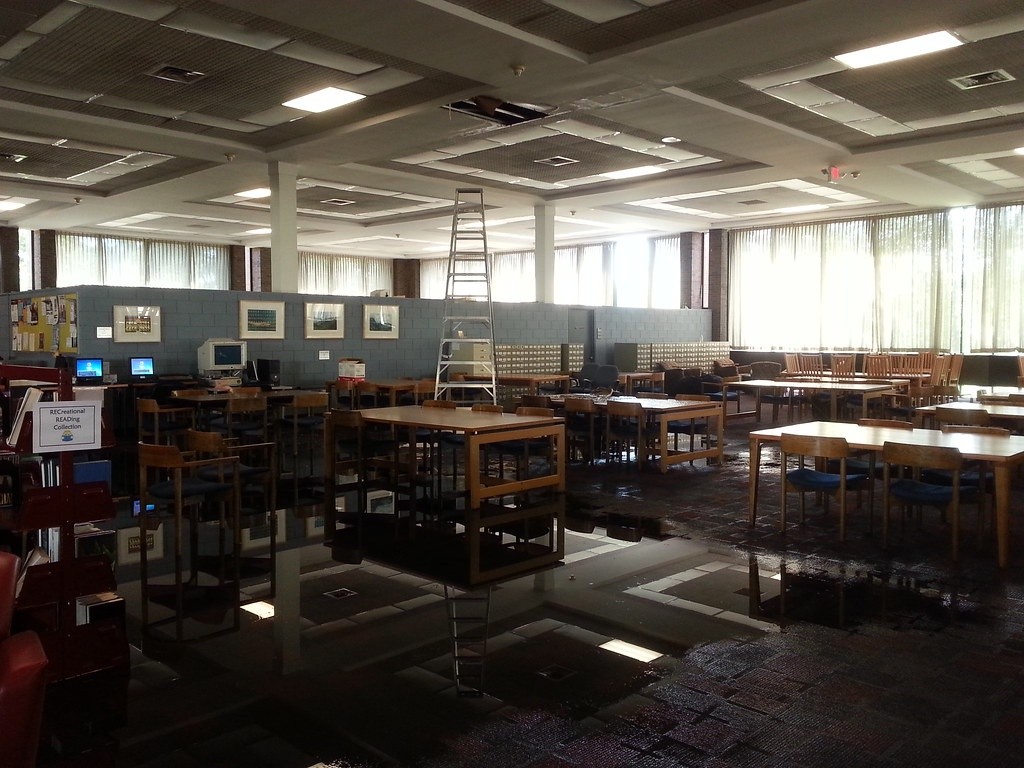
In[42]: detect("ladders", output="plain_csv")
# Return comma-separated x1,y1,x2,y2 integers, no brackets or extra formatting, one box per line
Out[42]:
433,187,505,406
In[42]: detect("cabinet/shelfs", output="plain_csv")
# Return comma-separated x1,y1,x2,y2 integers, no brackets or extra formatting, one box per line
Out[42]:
35,668,132,768
0,362,130,696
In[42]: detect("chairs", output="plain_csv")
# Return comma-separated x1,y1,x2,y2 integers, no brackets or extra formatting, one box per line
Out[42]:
182,516,276,625
139,349,1024,555
141,539,240,647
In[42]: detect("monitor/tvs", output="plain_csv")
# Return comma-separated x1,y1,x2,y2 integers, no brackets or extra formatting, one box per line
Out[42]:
75,359,103,381
129,358,154,379
197,341,247,372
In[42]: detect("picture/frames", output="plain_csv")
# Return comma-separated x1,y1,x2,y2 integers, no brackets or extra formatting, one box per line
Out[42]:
304,496,346,539
367,489,401,518
362,304,401,340
303,301,345,340
239,509,287,553
113,304,161,342
116,522,164,568
239,301,285,340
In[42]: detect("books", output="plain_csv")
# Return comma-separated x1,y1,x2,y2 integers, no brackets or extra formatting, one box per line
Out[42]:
1,450,125,636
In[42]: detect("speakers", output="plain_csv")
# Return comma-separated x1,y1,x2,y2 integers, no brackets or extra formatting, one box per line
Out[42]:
257,359,280,387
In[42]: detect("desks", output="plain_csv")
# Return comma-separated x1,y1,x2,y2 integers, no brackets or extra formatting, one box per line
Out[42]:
323,405,566,510
325,378,430,432
723,379,895,431
748,422,1024,569
915,401,1024,490
512,393,724,475
556,371,652,392
463,373,570,395
783,371,946,408
170,390,329,470
748,554,943,633
9,377,76,429
323,492,566,593
981,393,1024,405
40,383,128,402
